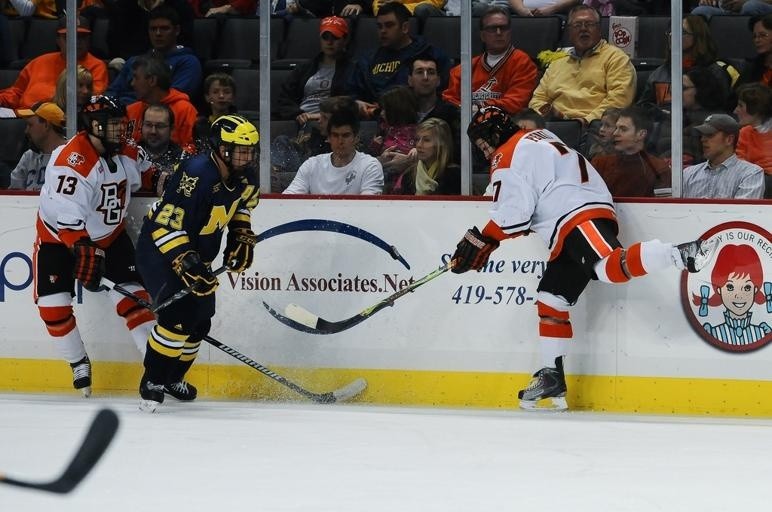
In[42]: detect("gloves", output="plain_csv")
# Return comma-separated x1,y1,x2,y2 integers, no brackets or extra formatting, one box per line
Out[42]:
470,237,500,271
451,226,486,274
222,227,256,272
72,237,106,291
171,250,219,297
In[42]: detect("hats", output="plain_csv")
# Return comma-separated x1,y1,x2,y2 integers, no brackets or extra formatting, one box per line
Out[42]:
319,16,349,39
693,114,739,135
15,101,67,129
56,15,92,34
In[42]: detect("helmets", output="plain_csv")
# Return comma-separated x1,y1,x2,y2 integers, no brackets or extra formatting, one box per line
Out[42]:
80,94,127,149
467,106,520,162
208,116,260,182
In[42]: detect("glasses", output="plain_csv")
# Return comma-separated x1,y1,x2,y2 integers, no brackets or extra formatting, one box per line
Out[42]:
752,33,770,40
142,120,168,130
570,21,597,28
681,83,694,91
683,26,693,37
480,24,511,33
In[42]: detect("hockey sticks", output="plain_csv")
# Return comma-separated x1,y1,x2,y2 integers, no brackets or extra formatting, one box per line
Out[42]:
150,262,231,315
0,409,120,494
285,257,466,333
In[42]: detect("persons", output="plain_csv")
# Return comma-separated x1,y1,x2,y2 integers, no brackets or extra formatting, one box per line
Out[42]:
363,85,419,162
391,118,460,197
0,16,109,110
283,108,384,196
529,5,633,129
407,57,461,167
683,113,765,201
692,245,772,346
133,101,193,176
509,108,545,130
279,16,352,119
452,106,720,411
7,101,66,191
134,112,259,407
296,95,359,166
637,16,716,106
191,74,237,144
127,57,199,147
731,13,772,88
589,107,672,198
442,8,539,117
645,64,711,165
510,0,583,18
52,65,94,112
586,106,622,161
734,82,769,201
340,2,451,120
32,94,171,398
105,11,201,97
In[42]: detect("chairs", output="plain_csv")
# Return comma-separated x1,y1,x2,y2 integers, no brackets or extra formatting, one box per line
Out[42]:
251,120,299,138
628,17,671,68
0,70,21,88
350,18,421,57
0,117,28,188
509,16,562,66
234,69,295,118
192,19,218,59
587,119,657,160
29,20,110,58
710,14,761,60
543,120,581,154
209,18,285,73
560,18,609,47
422,17,483,56
304,121,377,154
286,18,351,59
0,18,29,68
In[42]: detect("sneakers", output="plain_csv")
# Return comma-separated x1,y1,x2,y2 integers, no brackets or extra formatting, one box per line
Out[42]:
517,354,568,401
672,239,703,274
163,381,198,402
139,372,165,404
69,354,92,390
